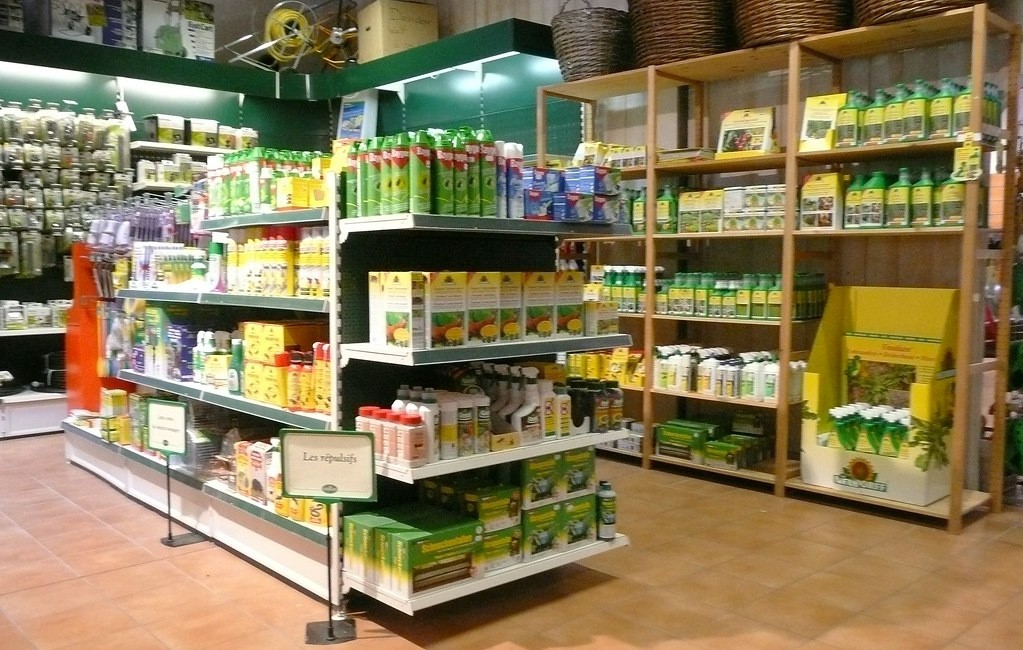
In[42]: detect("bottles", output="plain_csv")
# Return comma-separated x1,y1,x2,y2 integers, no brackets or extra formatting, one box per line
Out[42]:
355,361,623,469
601,266,825,321
623,185,677,234
285,341,330,416
208,126,524,217
844,168,985,229
192,330,245,396
655,344,807,404
834,75,1001,149
596,481,616,541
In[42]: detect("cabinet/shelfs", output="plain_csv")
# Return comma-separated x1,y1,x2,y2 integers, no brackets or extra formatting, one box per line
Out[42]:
61,208,633,639
0,140,240,438
536,5,1023,536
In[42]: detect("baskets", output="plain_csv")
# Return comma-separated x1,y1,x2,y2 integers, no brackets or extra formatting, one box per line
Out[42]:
550,0,632,83
627,0,732,70
855,0,1008,26
732,0,851,50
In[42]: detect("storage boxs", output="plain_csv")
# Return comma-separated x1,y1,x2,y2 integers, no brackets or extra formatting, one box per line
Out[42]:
653,412,771,470
365,270,619,350
356,0,439,65
341,441,600,599
240,319,329,409
